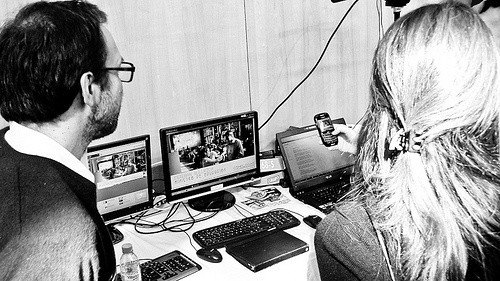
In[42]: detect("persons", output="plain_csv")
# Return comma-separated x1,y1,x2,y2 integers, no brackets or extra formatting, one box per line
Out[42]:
180,123,254,168
315,0,500,281
0,0,135,281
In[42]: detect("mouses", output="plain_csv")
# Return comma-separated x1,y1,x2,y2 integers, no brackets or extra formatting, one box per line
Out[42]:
303,215,323,229
196,248,222,263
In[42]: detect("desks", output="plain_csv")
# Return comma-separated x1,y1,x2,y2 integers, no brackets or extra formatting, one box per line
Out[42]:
112,184,328,281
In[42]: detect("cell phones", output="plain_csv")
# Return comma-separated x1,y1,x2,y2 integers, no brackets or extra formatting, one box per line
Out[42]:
314,113,338,146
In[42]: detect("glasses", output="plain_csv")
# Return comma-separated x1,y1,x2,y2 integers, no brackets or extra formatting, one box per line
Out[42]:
84,61,136,82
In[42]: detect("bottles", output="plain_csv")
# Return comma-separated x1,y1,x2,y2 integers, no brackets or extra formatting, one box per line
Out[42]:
120,243,142,281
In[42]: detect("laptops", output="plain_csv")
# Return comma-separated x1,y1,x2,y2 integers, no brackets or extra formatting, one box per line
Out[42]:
277,118,359,214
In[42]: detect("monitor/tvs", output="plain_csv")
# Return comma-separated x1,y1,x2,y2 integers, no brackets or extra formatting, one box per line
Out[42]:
159,109,260,212
86,136,153,245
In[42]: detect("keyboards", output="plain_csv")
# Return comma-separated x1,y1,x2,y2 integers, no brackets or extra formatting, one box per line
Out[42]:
194,211,301,249
114,251,203,281
